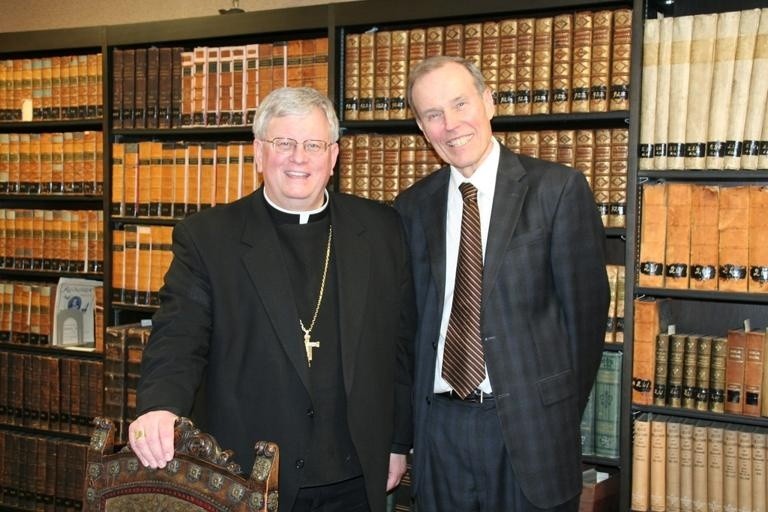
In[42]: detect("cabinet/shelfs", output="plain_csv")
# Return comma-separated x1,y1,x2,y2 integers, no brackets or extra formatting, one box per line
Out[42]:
619,0,767,512
100,1,341,418
323,1,627,509
1,23,116,511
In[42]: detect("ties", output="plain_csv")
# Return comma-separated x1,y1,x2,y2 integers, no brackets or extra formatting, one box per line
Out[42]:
441,183,486,400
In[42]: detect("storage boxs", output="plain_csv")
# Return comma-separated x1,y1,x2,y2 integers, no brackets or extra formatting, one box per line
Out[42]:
577,468,616,512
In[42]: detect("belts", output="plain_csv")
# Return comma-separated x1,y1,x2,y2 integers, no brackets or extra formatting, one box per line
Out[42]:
435,393,496,404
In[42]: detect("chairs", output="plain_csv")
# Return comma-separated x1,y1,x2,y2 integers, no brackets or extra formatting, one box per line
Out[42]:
78,411,280,512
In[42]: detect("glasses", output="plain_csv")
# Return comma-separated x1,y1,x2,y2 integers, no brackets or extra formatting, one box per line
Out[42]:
261,137,333,157
